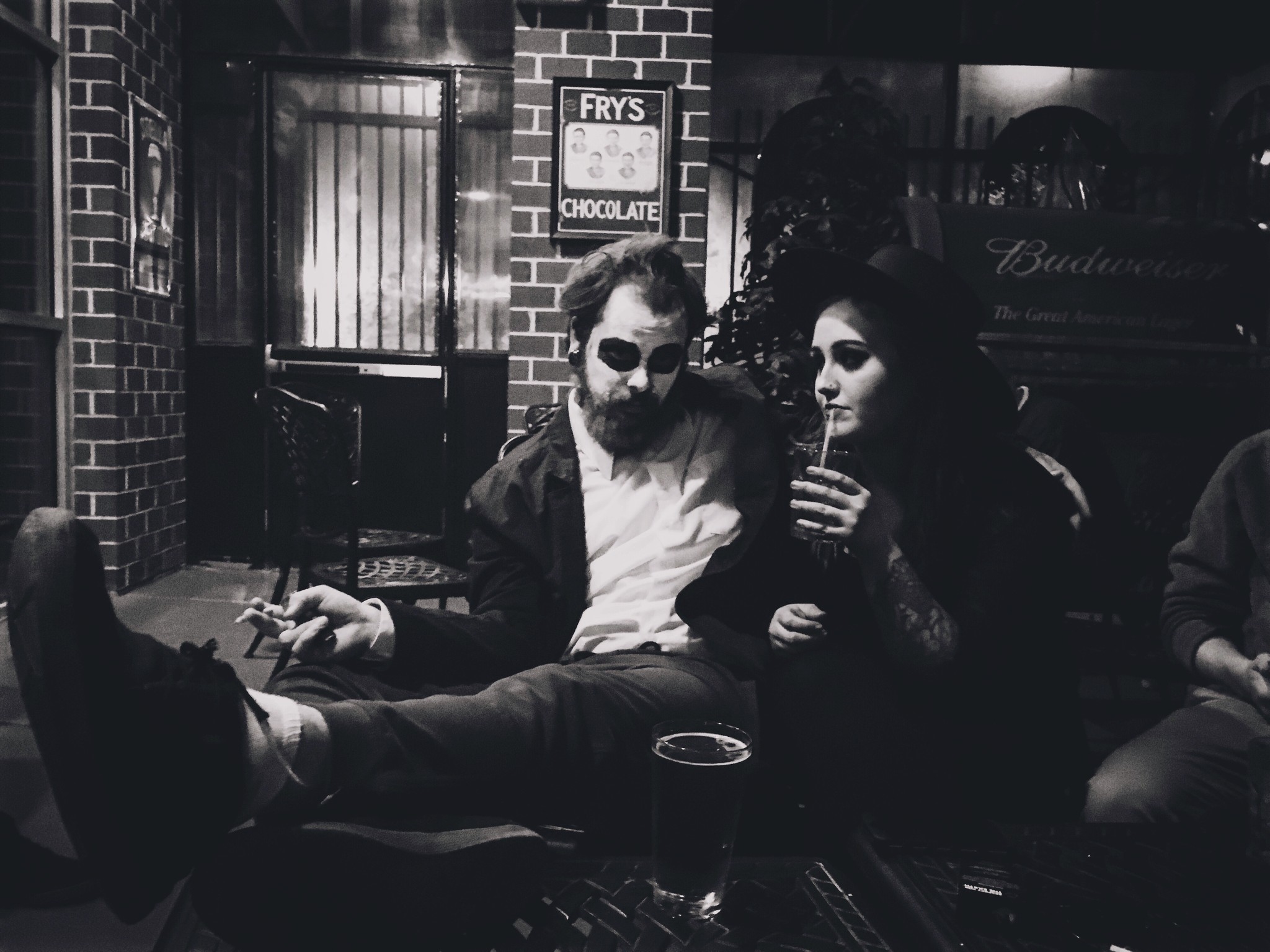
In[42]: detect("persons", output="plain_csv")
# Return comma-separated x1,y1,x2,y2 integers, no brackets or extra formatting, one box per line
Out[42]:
8,233,1090,924
571,127,655,180
673,245,1129,855
1087,414,1269,820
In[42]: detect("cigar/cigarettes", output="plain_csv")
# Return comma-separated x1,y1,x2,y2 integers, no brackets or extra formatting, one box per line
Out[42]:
232,607,273,628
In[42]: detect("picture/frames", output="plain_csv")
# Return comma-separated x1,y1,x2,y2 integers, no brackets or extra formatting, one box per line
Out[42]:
545,77,678,252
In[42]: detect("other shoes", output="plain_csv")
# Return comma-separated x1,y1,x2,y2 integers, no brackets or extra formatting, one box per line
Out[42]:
5,504,272,925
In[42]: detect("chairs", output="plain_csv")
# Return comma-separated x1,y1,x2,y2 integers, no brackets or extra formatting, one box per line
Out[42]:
263,385,473,695
236,344,450,663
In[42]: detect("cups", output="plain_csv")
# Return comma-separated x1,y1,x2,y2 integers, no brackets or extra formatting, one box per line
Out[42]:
651,719,753,920
790,447,856,564
